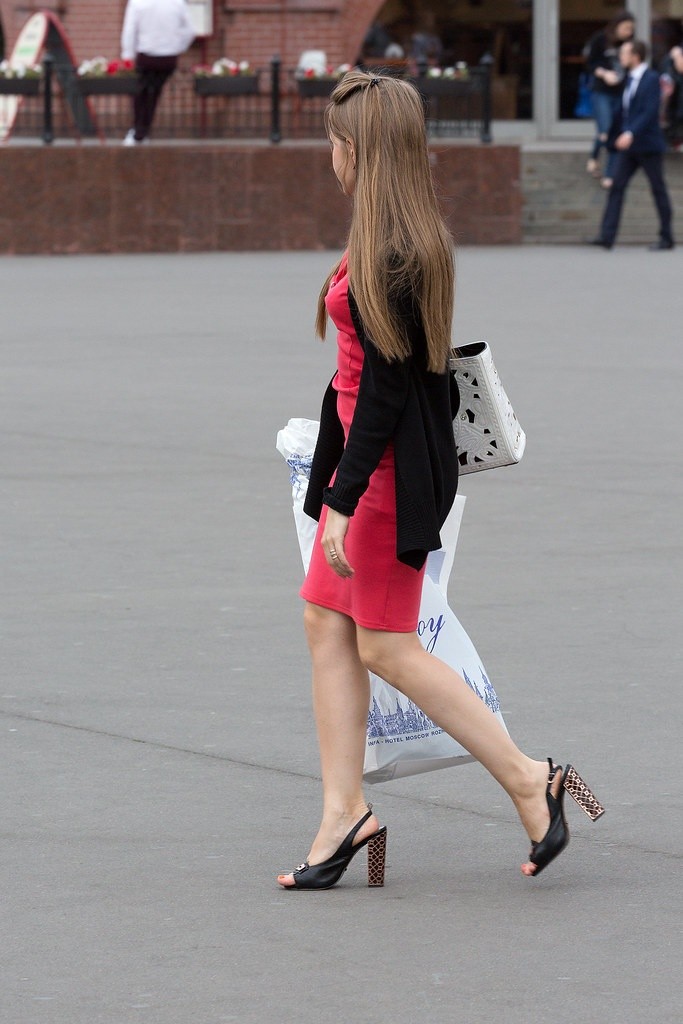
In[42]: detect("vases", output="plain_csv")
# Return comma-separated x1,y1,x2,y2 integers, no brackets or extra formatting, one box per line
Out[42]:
81,78,137,94
0,78,40,97
195,77,257,95
299,79,338,98
420,75,483,94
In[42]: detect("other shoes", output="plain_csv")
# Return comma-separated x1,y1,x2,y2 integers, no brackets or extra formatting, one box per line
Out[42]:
587,239,612,248
124,129,149,147
659,239,673,248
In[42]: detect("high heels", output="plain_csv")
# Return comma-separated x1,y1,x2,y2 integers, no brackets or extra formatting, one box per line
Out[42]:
529,758,606,876
283,802,386,890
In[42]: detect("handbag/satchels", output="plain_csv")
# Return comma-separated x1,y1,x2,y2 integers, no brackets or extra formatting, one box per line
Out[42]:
576,73,595,116
448,341,525,476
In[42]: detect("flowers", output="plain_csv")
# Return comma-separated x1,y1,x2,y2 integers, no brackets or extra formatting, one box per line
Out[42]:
185,57,255,78
73,53,138,77
0,59,45,78
425,61,471,81
291,61,351,80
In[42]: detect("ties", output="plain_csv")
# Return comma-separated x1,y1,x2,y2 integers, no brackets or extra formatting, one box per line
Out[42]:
626,77,632,90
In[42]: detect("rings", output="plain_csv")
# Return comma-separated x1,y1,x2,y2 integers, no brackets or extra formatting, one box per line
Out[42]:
330,549,338,559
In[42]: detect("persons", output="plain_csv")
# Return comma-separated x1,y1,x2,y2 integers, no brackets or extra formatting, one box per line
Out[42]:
578,13,683,188
276,69,606,889
589,40,673,250
119,0,196,146
